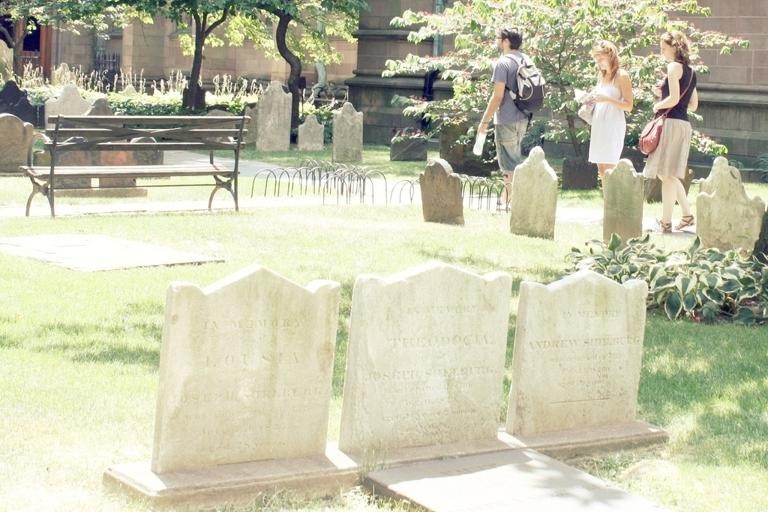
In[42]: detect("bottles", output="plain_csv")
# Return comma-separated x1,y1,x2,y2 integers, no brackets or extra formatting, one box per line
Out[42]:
472,131,488,156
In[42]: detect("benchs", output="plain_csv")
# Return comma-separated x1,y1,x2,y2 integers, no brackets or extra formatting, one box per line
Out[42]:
17,114,251,217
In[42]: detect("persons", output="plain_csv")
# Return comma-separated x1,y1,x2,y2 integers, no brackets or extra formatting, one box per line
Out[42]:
647,31,699,234
580,39,634,194
476,26,534,213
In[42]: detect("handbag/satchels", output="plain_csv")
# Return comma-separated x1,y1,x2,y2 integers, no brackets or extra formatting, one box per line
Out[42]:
637,111,667,155
577,100,597,126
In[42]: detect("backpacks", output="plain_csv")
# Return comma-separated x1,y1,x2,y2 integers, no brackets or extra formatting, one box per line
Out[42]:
499,51,546,114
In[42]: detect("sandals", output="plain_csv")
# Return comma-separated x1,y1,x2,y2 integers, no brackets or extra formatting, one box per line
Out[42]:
646,220,673,234
674,213,695,231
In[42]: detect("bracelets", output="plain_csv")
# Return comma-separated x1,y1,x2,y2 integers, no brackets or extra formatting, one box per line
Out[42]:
480,120,490,125
